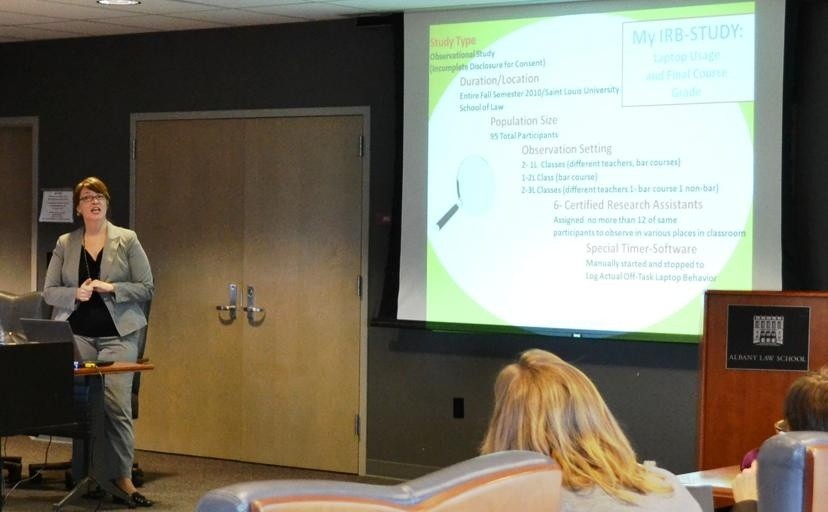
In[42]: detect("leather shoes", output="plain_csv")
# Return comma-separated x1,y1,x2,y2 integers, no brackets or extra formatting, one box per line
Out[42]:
112,483,151,506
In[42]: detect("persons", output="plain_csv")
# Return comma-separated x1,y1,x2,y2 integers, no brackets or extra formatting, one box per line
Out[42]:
44,175,156,507
479,349,705,512
730,369,828,512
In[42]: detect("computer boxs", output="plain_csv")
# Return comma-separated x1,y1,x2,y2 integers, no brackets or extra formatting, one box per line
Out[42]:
0,341,74,435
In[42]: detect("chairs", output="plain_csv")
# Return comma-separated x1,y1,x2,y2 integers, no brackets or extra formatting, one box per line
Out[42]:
1,288,152,486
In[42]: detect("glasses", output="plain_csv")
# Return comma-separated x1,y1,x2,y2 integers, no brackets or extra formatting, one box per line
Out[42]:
79,194,105,201
773,420,787,434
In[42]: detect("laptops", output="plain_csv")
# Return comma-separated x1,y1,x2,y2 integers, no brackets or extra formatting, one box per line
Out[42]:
19,318,115,368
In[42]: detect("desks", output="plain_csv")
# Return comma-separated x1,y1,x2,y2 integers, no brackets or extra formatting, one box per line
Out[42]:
48,359,154,507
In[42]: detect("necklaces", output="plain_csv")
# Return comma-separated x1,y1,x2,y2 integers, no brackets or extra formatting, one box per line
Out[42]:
82,235,90,282
